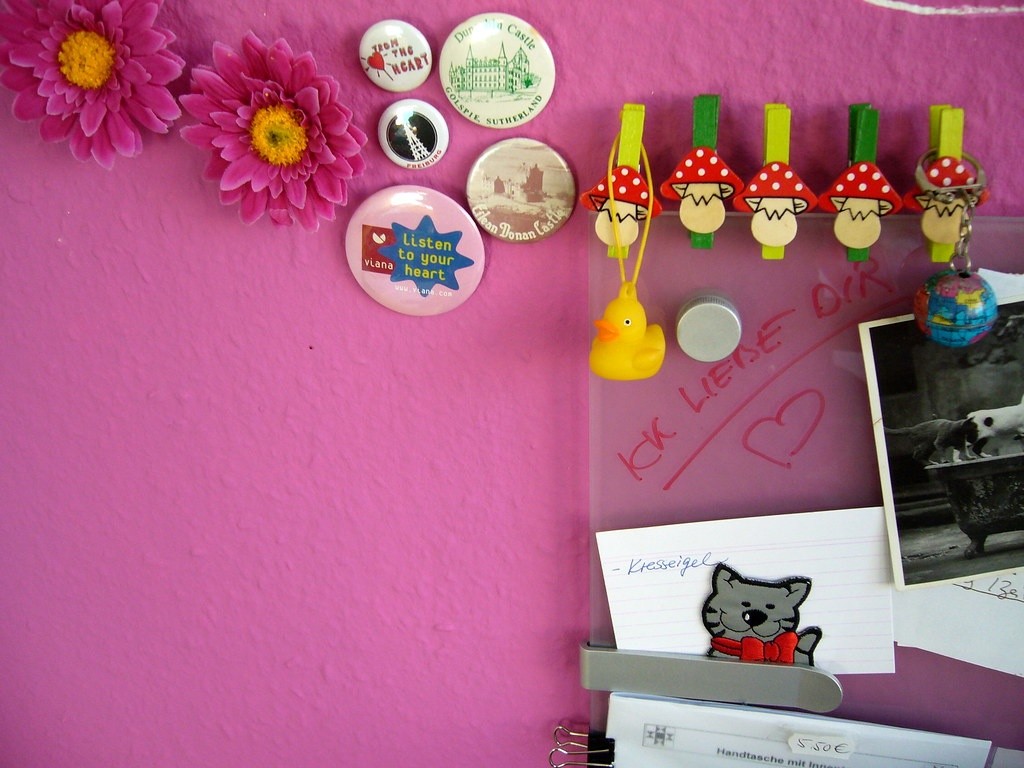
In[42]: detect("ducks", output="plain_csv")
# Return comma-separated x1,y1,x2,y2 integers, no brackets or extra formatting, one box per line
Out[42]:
588,298,665,382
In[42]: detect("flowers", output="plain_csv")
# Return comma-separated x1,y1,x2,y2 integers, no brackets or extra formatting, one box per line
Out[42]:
178,26,369,234
0,0,188,173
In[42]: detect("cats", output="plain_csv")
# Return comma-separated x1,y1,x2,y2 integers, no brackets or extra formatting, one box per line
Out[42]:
881,394,1024,465
